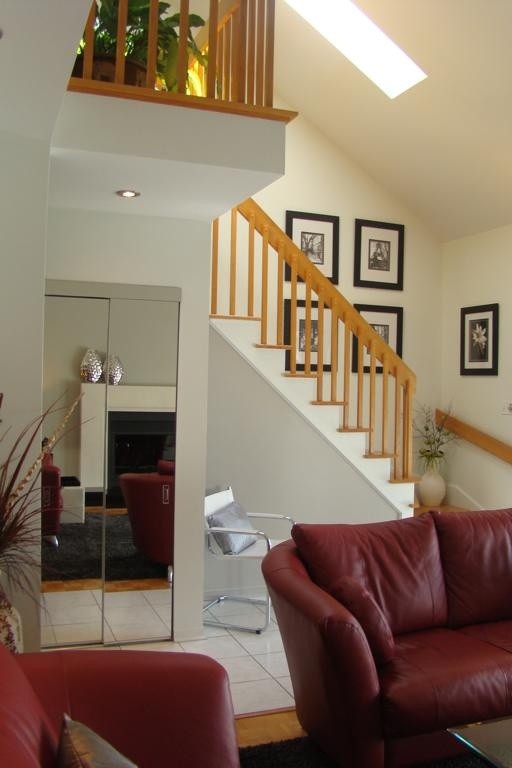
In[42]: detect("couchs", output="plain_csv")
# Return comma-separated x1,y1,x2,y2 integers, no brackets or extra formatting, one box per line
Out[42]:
250,495,511,767
0,638,238,766
111,454,174,577
39,447,65,549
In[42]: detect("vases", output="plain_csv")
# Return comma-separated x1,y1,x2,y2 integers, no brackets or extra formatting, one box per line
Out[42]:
79,347,103,383
102,354,125,386
0,595,26,653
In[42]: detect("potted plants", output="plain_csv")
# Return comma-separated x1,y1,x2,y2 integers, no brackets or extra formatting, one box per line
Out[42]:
410,403,461,507
62,1,209,101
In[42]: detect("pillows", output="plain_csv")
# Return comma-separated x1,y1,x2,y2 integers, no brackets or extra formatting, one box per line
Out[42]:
54,709,142,767
327,573,396,667
289,509,449,635
425,507,511,629
206,500,260,554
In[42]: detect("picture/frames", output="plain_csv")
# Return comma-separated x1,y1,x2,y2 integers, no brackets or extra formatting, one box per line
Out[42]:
456,298,501,376
281,295,338,374
349,300,406,374
349,215,408,293
279,207,340,287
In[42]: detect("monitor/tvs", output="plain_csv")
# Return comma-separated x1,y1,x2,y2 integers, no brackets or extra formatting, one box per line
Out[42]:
112,434,164,476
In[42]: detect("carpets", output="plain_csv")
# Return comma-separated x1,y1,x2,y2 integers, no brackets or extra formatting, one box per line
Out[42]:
43,506,163,583
238,730,501,768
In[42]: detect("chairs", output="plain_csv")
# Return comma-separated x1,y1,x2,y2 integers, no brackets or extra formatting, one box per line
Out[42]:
203,482,297,636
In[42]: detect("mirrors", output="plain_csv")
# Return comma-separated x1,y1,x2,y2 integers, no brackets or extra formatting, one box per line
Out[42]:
43,276,182,648
